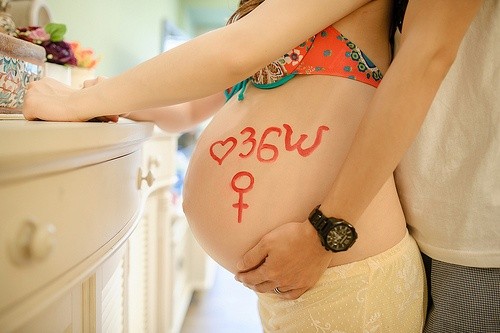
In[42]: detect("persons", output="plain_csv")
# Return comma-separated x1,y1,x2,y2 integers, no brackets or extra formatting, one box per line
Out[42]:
235,0,500,333
22,0,427,333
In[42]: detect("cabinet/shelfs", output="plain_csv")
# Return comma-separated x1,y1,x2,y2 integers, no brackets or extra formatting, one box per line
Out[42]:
0,119,213,333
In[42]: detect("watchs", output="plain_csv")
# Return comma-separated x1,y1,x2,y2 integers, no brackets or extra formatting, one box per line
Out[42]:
308,204,358,253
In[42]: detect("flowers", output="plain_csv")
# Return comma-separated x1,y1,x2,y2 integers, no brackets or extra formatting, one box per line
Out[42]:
68,40,102,68
17,22,77,65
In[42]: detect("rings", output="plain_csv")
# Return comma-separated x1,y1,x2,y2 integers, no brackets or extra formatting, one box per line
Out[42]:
273,288,281,295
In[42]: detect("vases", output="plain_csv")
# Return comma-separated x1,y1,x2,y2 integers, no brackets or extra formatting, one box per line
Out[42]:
70,67,93,89
47,64,72,88
